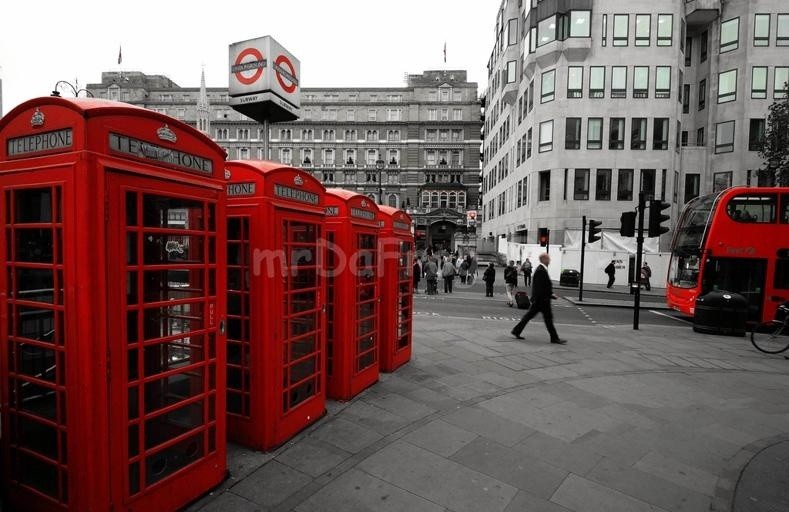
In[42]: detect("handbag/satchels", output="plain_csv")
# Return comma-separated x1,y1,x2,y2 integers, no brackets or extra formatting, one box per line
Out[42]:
482,275,487,282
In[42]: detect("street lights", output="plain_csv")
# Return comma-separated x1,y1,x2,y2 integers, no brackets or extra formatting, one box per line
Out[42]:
376,159,384,205
50,76,95,98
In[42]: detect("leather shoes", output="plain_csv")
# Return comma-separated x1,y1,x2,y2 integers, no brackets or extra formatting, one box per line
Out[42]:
550,339,568,345
511,331,525,340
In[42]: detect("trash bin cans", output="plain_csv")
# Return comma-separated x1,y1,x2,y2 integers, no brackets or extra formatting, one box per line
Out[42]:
630,282,639,295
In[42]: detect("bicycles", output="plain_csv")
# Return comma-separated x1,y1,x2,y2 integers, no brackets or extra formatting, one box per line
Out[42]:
750,304,789,354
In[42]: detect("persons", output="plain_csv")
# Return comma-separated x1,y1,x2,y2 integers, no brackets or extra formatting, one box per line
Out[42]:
604,260,616,288
503,260,518,307
481,262,496,296
515,261,522,276
522,258,532,286
510,253,568,345
412,242,478,295
641,261,651,290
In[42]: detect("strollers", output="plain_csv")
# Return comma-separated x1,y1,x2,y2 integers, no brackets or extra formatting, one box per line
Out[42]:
425,278,439,294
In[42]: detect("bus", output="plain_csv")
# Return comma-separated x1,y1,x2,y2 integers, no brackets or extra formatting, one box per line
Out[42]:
666,186,789,336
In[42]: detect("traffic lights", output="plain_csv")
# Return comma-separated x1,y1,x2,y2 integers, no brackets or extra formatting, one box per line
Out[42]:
589,219,602,243
648,202,671,236
620,211,635,236
538,227,547,247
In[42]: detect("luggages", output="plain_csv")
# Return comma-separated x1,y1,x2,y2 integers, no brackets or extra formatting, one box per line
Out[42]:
515,291,531,310
